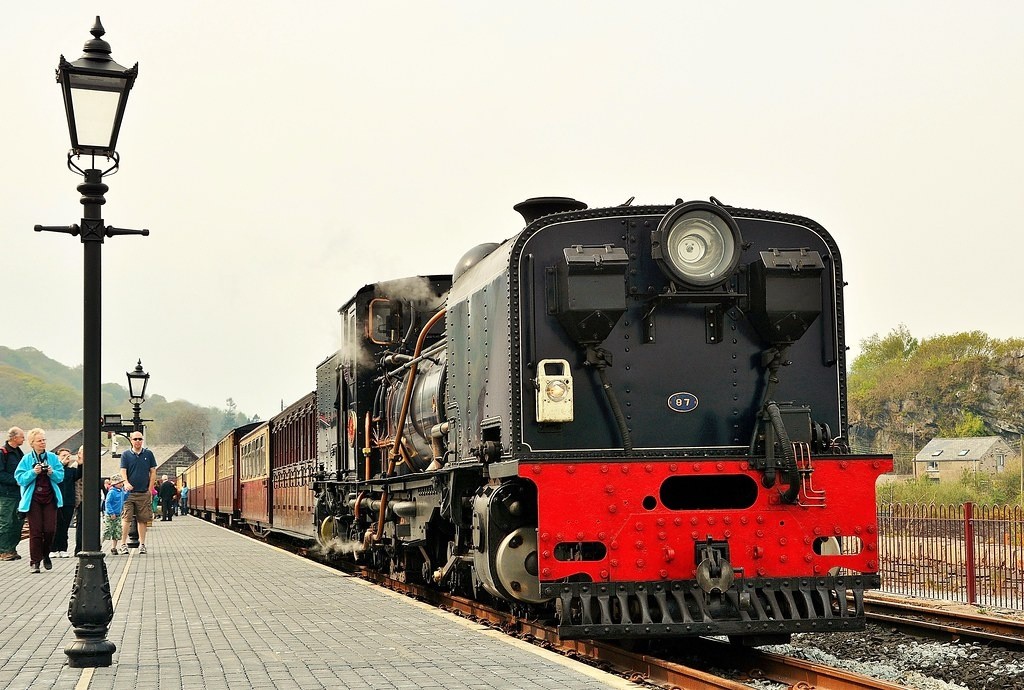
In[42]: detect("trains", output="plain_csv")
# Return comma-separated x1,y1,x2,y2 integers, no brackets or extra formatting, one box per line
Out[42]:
177,194,896,653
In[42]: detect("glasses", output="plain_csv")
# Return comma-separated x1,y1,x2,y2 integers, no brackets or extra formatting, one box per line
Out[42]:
132,438,143,441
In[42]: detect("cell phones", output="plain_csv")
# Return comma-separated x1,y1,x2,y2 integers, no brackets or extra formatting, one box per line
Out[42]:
69,455,78,460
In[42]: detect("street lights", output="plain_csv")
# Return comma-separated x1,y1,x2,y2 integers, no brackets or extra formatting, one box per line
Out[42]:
126,359,149,448
34,16,149,670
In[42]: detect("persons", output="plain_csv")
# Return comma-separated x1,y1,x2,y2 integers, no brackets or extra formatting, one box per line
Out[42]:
48,445,189,559
117,431,158,554
14,427,65,572
0,426,25,561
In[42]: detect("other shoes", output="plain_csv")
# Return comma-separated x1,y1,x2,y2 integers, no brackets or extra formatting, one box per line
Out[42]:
10,551,22,559
111,548,118,555
119,544,129,554
42,556,53,570
138,545,147,553
29,560,40,573
60,551,70,558
0,553,15,560
49,551,59,558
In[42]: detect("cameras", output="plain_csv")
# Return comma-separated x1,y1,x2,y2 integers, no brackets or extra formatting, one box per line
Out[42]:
38,452,49,472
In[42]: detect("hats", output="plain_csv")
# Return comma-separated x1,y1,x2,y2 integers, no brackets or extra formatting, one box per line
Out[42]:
110,473,126,485
161,475,168,481
